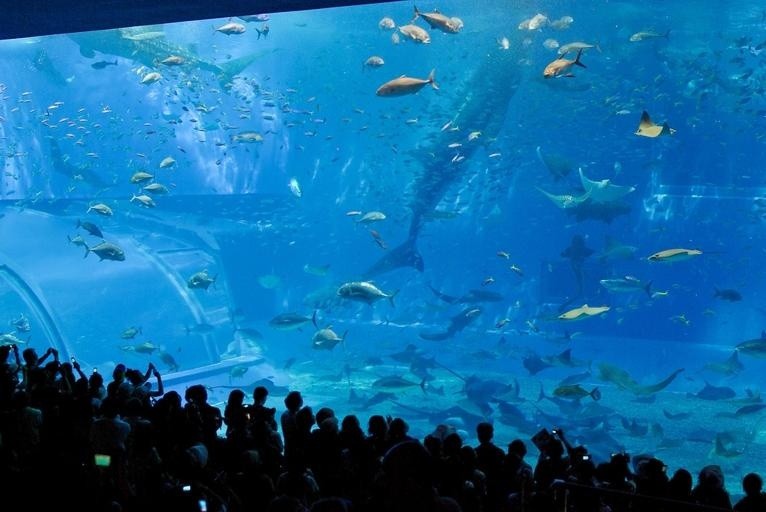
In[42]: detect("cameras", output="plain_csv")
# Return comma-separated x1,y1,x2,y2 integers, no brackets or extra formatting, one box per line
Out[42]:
552,429,558,434
94,452,112,466
182,483,192,491
582,454,592,460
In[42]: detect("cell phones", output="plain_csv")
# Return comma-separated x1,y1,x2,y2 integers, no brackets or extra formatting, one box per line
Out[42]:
198,500,207,511
242,404,249,408
71,357,76,366
93,367,98,375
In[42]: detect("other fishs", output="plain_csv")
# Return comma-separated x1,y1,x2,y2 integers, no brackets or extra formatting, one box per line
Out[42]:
0,4,766,465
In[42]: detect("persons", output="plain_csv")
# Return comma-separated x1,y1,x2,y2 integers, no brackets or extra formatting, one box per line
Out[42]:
0,344,766,512
